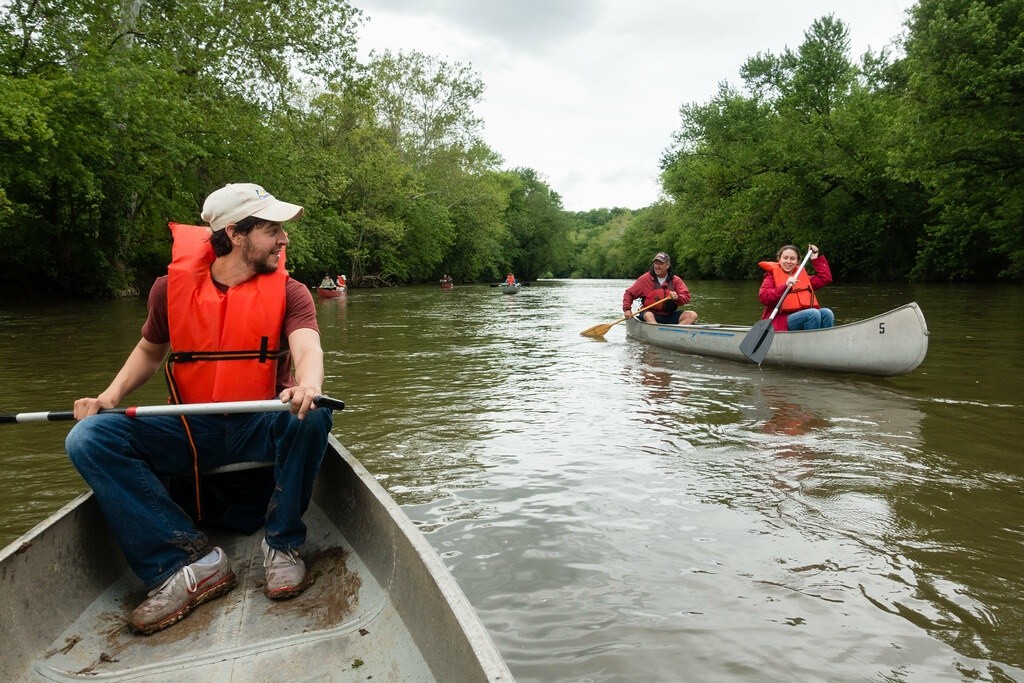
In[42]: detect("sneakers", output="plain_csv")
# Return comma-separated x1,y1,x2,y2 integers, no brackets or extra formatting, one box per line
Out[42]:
261,537,309,599
129,547,237,633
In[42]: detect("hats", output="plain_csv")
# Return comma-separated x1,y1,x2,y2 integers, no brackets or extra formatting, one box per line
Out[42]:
201,183,304,232
342,275,346,280
654,251,670,263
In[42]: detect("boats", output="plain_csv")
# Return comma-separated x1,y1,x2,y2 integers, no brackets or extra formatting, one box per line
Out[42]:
317,286,347,297
441,282,453,288
500,282,521,294
625,296,930,377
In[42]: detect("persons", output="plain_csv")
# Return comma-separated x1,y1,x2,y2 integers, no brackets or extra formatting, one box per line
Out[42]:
758,243,834,332
319,273,336,288
335,275,347,291
504,271,518,286
623,252,698,324
66,182,334,637
440,274,452,285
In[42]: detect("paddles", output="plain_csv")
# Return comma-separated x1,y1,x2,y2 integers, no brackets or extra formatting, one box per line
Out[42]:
490,282,530,287
0,395,345,424
739,247,819,364
309,286,319,288
580,294,672,337
